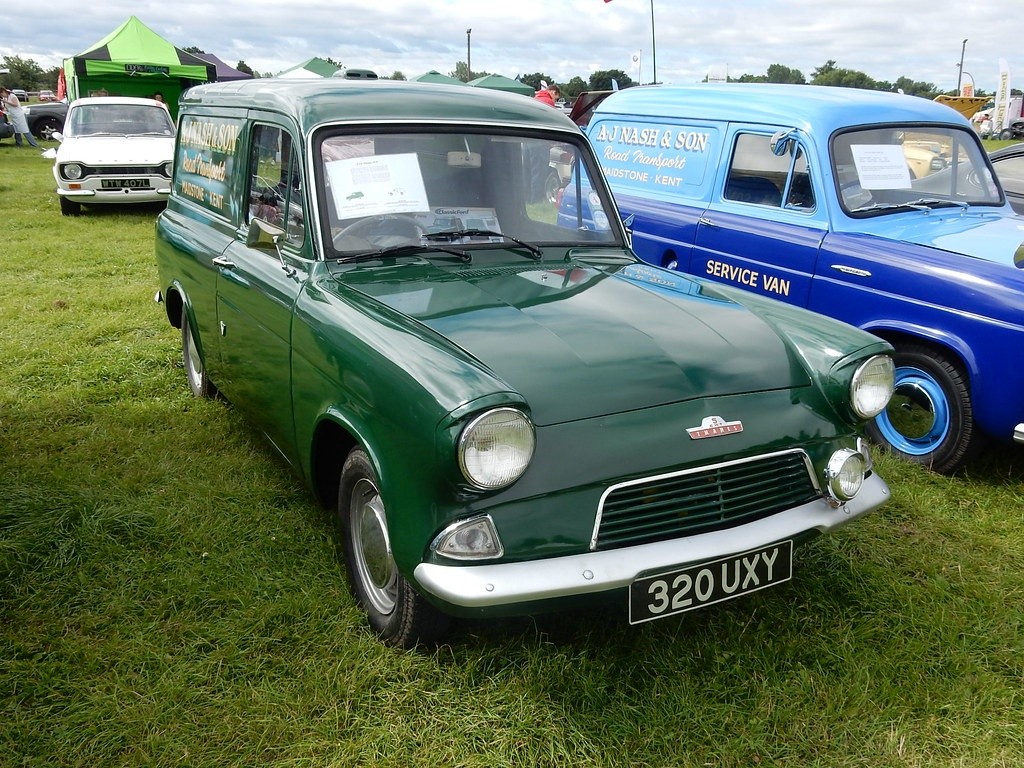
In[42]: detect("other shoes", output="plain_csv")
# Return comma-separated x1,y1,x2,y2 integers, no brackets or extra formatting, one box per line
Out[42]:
526,196,547,203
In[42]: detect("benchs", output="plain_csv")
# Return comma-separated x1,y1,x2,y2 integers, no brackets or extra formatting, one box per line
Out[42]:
75,121,163,134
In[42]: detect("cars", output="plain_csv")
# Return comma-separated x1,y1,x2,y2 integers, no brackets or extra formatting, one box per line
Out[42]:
388,187,406,196
523,89,620,205
908,140,1024,215
321,135,374,188
895,94,996,180
23,101,69,142
553,79,1024,480
346,191,364,200
0,109,15,141
40,96,178,217
426,215,465,242
149,73,897,651
38,89,58,102
12,88,30,103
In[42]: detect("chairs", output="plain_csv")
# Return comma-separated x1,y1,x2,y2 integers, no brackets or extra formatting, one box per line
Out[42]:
726,175,781,207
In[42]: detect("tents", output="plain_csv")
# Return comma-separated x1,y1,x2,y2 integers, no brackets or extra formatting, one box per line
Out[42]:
57,16,535,131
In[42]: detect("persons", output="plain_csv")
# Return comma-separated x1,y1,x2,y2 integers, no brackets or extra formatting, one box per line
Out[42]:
520,85,560,205
0,87,38,147
154,92,170,111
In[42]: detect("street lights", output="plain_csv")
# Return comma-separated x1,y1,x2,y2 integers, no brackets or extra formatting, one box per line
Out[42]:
466,28,472,81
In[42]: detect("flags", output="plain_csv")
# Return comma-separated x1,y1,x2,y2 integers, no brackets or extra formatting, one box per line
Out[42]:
631,50,640,70
